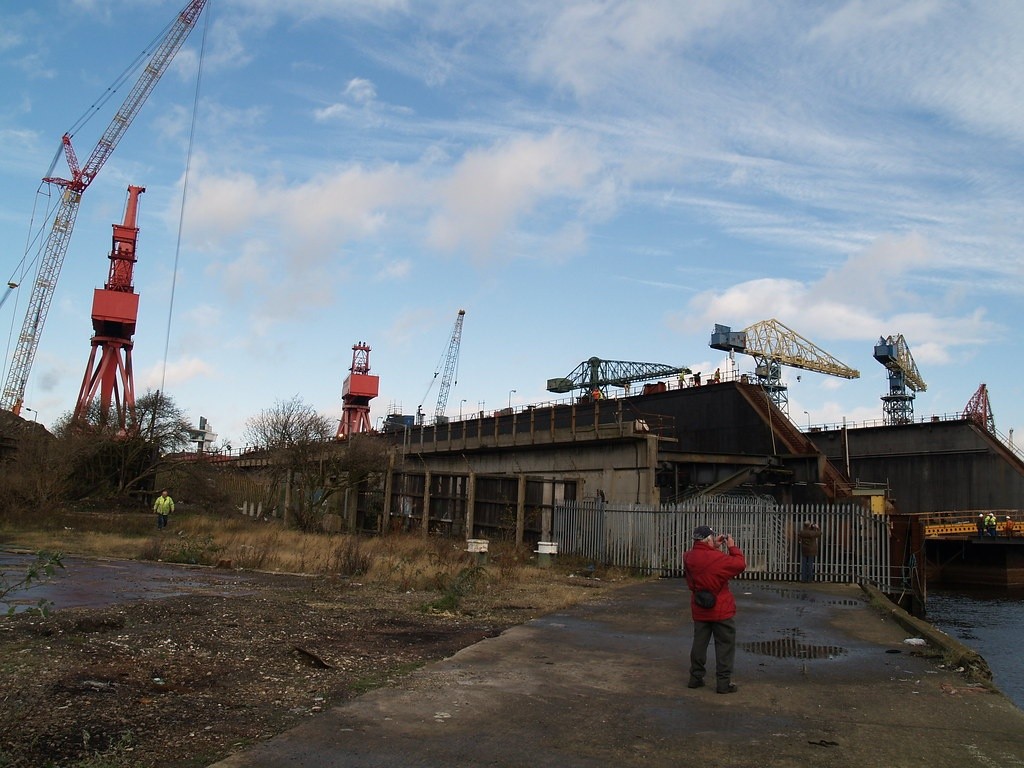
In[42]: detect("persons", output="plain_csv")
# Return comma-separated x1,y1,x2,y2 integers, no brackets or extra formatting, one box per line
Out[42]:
683,525,746,693
985,511,994,536
989,513,996,536
976,514,985,536
694,372,701,386
714,368,720,383
678,371,685,389
1003,516,1014,536
799,520,822,583
592,387,603,402
153,491,174,531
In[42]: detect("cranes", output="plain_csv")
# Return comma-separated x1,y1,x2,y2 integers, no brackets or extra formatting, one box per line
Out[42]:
333,340,380,438
0,0,215,443
415,309,466,426
707,319,860,420
874,332,927,425
546,356,693,400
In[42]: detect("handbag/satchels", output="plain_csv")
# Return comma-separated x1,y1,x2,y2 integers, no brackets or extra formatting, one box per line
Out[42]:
693,588,716,610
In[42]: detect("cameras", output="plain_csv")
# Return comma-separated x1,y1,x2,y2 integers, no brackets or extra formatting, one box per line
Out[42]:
722,534,728,543
811,524,815,528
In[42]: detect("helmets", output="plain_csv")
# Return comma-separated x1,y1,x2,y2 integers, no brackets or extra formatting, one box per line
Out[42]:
989,513,994,518
1006,516,1010,519
979,514,983,517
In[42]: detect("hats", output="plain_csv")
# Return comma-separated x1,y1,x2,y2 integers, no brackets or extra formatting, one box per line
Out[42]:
692,525,717,540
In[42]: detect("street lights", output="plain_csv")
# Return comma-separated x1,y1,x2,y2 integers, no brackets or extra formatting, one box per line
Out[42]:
26,407,38,437
459,399,467,420
509,389,517,408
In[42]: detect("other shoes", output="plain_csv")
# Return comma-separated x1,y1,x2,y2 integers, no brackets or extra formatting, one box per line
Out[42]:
716,684,737,694
688,678,705,688
